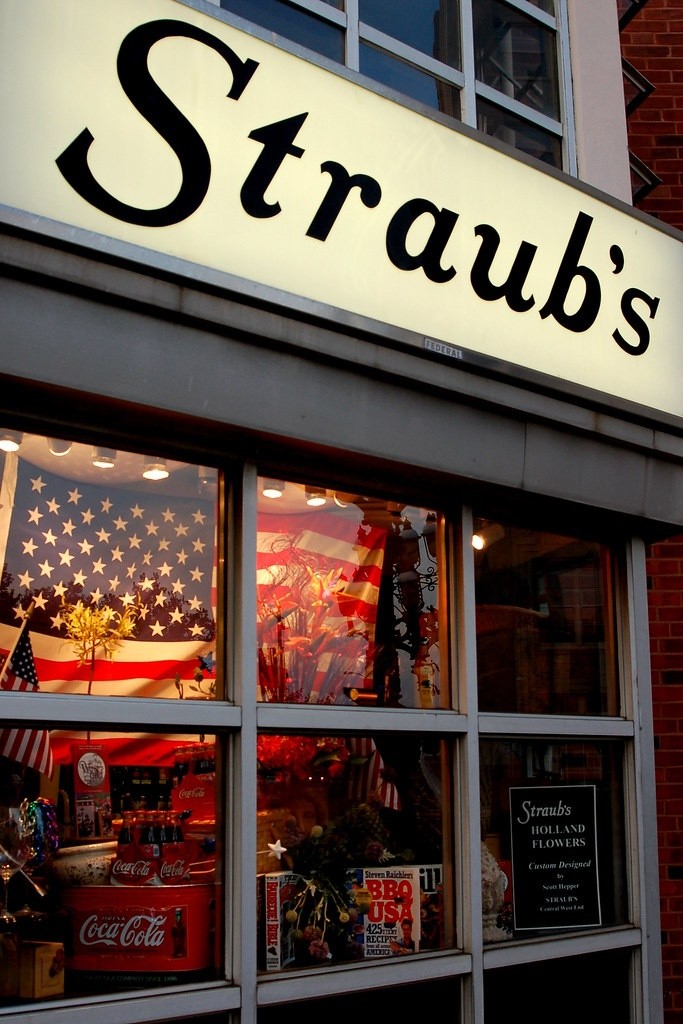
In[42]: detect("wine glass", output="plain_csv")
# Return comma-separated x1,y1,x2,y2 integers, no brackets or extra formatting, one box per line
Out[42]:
0,818,31,926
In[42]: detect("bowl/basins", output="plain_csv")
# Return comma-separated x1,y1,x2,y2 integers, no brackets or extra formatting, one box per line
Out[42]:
54,841,118,885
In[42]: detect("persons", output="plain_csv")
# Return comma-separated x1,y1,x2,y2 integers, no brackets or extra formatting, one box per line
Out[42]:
269,790,325,842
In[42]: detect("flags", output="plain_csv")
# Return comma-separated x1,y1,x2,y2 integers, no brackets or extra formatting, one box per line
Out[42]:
344,738,401,811
1,624,55,777
1,448,388,777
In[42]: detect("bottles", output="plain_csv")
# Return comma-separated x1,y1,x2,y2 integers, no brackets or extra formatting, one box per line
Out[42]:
116,811,186,884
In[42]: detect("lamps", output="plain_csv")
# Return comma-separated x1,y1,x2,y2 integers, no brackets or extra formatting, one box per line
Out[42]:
91,446,115,469
333,490,360,507
305,486,327,507
0,428,24,451
262,476,285,498
46,438,72,456
141,455,170,480
473,520,505,551
